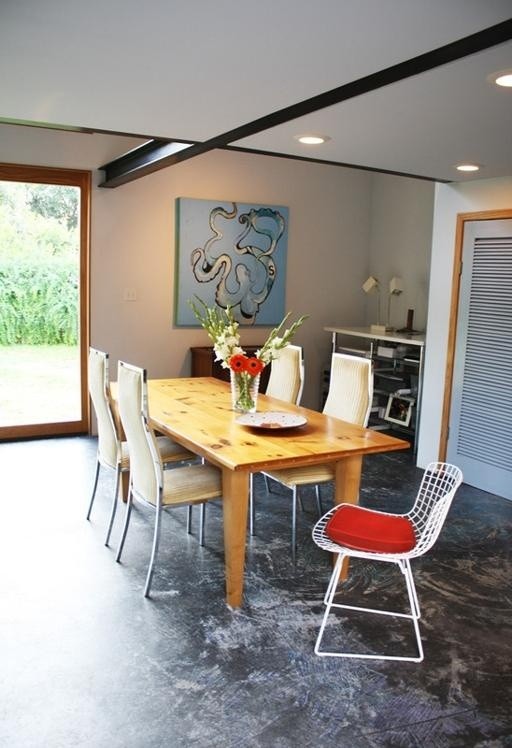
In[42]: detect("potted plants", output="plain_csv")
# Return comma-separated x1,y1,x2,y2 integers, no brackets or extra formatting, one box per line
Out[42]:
383,394,415,427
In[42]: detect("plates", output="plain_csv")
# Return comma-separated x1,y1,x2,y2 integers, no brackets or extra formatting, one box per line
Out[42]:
323,325,426,455
188,345,272,395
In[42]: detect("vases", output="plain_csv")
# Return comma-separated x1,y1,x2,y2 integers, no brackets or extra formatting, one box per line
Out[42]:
185,293,312,409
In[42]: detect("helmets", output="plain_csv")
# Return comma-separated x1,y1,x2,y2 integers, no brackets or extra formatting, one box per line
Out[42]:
235,412,308,430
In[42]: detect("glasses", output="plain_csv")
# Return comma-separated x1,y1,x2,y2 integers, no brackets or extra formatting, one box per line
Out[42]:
361,276,404,333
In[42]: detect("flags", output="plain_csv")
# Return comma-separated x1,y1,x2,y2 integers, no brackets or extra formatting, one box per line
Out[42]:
229,368,261,415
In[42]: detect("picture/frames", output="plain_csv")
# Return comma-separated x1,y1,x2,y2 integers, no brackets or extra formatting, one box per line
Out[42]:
108,376,411,608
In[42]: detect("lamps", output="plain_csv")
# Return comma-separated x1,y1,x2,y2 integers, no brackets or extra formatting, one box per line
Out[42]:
262,338,306,494
85,345,197,547
311,460,464,662
115,358,223,598
249,351,376,567
